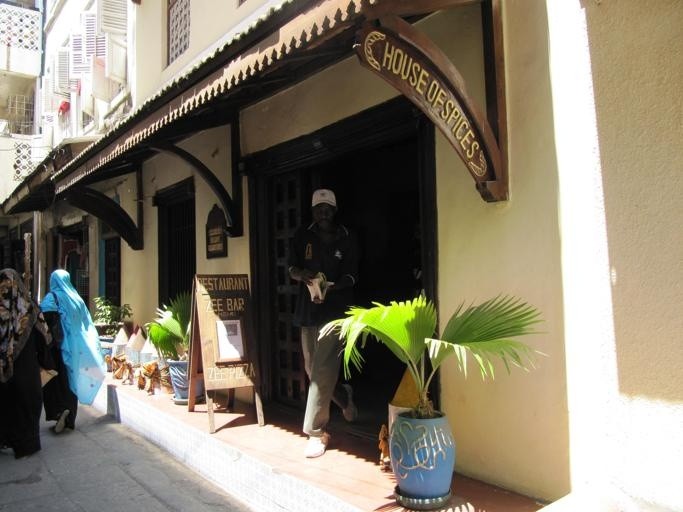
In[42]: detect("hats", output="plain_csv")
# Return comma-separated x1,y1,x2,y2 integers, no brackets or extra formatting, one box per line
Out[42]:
309,188,338,210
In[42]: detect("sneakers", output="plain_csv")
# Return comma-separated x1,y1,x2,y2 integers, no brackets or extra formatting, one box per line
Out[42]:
340,382,359,423
53,407,75,434
303,429,329,459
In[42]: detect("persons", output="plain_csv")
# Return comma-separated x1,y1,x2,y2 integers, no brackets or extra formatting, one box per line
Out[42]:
286,187,363,462
37,268,88,434
0,268,45,459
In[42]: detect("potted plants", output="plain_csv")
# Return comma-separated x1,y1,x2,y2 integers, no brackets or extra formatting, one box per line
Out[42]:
318,289,551,506
145,289,207,406
92,298,134,372
